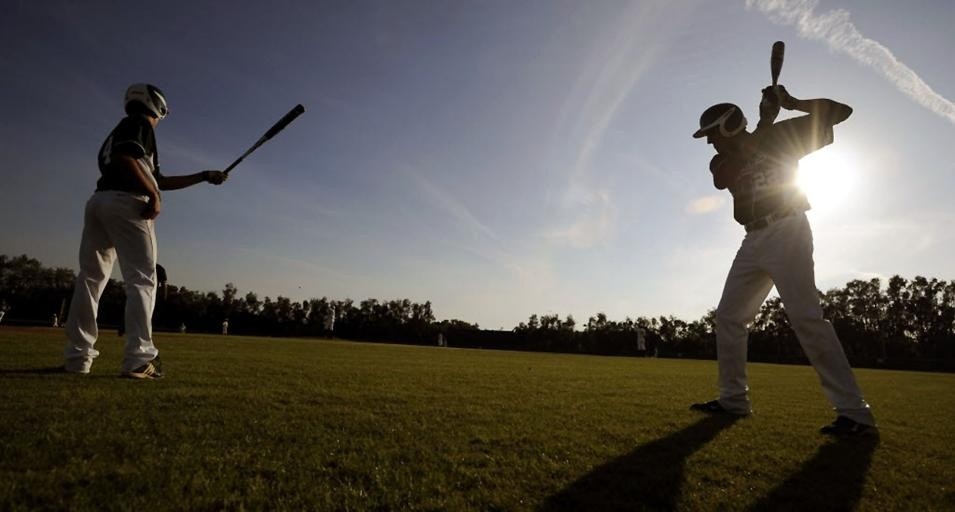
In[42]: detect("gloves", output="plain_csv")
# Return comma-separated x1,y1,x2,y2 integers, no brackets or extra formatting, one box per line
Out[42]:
761,83,800,111
758,91,781,123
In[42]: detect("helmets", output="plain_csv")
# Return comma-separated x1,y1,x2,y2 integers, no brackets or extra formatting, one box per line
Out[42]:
691,102,749,141
123,82,169,121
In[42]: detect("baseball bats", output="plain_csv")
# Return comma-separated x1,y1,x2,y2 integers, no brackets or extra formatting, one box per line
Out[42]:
224,104,305,180
770,41,785,99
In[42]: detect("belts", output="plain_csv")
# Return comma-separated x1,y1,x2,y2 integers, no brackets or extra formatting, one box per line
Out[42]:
745,204,806,233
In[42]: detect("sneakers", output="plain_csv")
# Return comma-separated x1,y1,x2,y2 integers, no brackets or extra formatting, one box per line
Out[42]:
64,356,94,373
126,360,162,380
819,415,871,437
688,397,730,413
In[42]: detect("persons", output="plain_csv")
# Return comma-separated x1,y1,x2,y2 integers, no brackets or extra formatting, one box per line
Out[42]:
322,303,337,341
52,313,58,327
221,318,229,335
689,84,882,443
60,83,228,382
